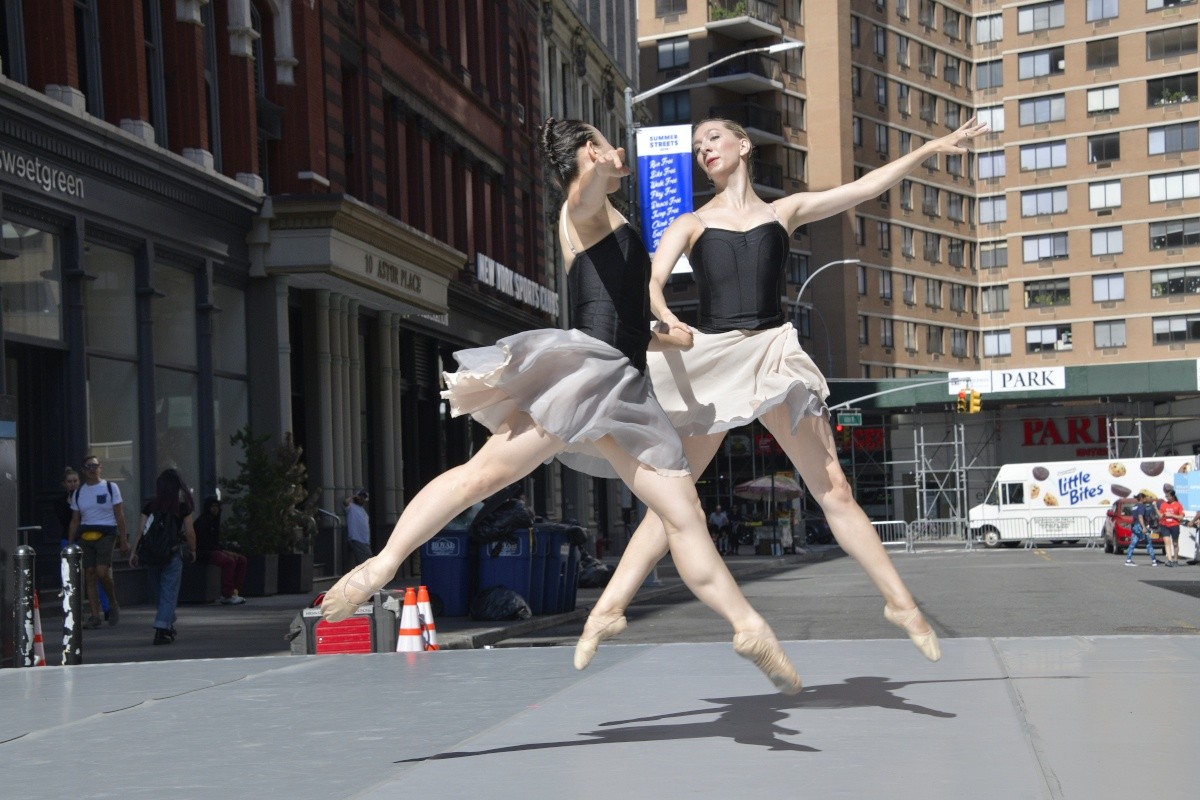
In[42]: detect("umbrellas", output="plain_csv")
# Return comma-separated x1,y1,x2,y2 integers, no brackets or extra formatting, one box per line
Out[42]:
733,473,805,519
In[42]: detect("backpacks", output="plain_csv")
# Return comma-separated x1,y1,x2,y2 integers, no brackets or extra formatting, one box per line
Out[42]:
138,516,177,572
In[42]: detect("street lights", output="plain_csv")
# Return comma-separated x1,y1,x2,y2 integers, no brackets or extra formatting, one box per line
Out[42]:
622,37,806,224
792,257,862,548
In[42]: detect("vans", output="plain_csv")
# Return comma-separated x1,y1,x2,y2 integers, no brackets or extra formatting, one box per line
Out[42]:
965,455,1200,549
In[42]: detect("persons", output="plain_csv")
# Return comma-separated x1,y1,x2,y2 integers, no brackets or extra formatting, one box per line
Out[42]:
1124,493,1158,566
1152,489,1184,567
129,468,197,646
54,455,131,630
571,116,990,673
342,490,372,568
709,503,741,556
193,494,248,605
321,116,804,698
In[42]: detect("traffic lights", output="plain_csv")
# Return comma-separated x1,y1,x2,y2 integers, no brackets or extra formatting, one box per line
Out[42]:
843,426,852,442
969,390,983,415
835,425,843,442
957,391,966,415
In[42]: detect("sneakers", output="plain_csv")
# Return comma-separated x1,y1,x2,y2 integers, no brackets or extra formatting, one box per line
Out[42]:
1151,559,1157,566
1125,560,1137,566
1166,561,1177,568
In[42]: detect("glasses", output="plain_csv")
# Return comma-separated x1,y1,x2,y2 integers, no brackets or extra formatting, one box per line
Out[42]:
84,463,101,470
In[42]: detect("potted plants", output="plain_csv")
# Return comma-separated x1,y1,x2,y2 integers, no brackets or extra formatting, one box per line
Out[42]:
205,425,322,596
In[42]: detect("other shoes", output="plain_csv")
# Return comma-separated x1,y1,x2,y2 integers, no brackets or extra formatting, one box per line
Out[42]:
83,615,101,628
109,604,120,624
220,594,246,603
154,626,176,645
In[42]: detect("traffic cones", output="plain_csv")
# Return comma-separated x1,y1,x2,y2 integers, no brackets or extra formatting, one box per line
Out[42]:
395,587,425,653
417,585,439,651
32,589,48,667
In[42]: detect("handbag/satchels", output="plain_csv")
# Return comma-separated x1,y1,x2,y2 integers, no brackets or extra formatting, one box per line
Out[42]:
1131,523,1146,540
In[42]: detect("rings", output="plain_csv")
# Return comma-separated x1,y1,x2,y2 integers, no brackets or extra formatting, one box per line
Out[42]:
965,132,969,138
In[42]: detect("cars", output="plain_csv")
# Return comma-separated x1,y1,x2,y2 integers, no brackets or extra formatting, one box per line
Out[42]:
1100,498,1166,556
736,507,835,545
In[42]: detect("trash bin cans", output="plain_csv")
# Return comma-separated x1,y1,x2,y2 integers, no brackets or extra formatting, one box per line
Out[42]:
418,529,473,617
474,514,590,614
